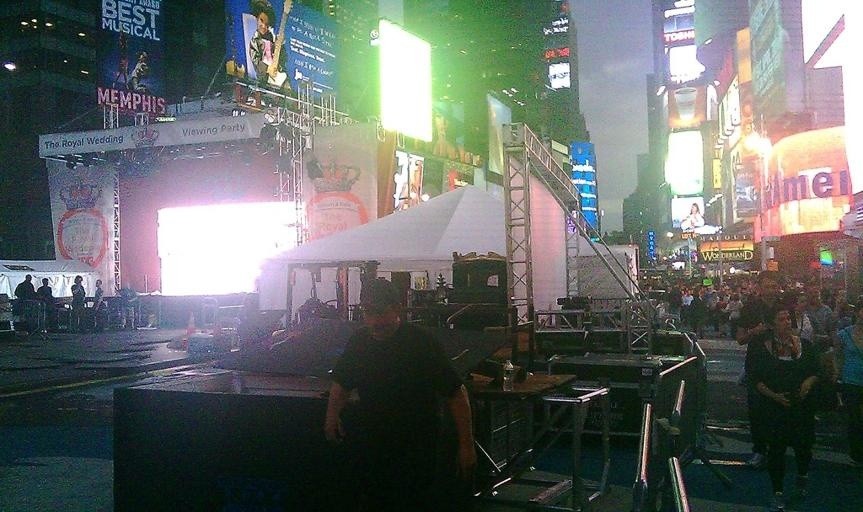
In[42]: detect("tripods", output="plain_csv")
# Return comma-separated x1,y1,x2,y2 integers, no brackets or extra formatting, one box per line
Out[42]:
27,303,54,341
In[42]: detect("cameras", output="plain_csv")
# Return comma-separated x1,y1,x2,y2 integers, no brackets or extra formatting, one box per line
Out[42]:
784,389,801,404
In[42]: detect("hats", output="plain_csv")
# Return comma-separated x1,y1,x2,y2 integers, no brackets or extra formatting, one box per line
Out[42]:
251,0,276,29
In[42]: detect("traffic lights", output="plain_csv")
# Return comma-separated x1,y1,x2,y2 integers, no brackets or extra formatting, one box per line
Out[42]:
810,255,820,269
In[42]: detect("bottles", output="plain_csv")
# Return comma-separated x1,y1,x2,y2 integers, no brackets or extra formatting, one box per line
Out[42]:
502,359,513,382
503,383,513,394
304,160,370,238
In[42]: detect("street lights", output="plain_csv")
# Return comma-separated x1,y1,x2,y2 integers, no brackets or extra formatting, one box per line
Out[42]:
680,220,694,280
667,230,673,277
713,225,723,285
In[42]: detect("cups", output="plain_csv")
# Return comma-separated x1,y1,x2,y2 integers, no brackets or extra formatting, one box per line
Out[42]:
674,87,697,121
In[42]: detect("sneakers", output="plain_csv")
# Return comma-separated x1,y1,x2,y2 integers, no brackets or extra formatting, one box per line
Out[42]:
746,452,767,470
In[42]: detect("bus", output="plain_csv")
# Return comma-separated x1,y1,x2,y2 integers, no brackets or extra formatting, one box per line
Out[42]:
638,267,686,283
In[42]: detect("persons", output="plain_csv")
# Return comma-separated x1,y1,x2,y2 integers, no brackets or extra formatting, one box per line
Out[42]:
71,276,85,334
433,102,464,161
15,275,35,300
129,50,151,94
681,202,704,230
112,27,129,91
116,282,137,331
325,278,477,509
38,278,56,309
91,279,106,331
237,293,353,381
249,2,293,107
